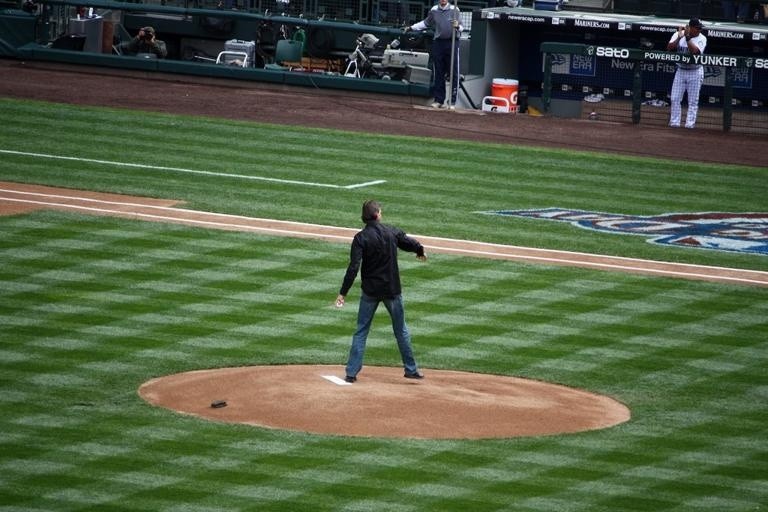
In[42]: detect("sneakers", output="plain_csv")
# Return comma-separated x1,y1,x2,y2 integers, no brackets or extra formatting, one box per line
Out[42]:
404,369,424,379
344,375,357,383
430,101,442,108
447,105,456,112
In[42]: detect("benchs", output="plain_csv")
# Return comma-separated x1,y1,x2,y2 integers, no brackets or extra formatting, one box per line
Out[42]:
580,100,768,136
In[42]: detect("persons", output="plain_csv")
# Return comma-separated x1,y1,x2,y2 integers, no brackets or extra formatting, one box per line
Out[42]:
400,1,464,112
336,200,428,382
669,20,709,130
128,26,168,61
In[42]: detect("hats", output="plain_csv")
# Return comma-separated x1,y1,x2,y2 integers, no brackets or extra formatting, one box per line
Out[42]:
689,17,705,27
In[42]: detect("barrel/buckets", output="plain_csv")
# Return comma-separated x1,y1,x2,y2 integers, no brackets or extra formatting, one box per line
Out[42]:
491,78,518,115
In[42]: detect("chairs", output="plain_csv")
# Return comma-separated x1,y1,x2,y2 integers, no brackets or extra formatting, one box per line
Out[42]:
264,39,303,71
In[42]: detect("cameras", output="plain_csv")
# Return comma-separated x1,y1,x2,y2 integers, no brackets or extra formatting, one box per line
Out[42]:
139,29,154,41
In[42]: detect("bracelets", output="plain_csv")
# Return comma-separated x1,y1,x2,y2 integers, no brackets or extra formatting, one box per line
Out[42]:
685,36,692,42
